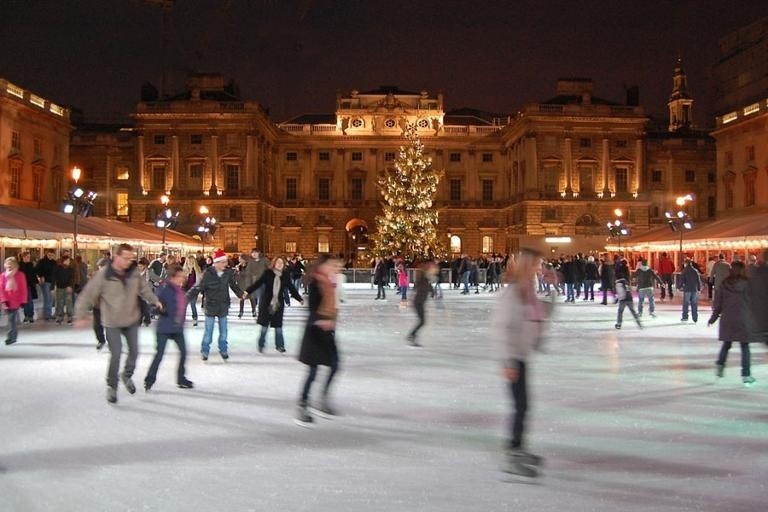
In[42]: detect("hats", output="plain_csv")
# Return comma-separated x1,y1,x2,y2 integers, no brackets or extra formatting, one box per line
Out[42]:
213,248,227,263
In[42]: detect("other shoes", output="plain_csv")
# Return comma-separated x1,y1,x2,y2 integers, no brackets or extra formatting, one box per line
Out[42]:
406,333,419,347
191,303,293,361
374,283,506,301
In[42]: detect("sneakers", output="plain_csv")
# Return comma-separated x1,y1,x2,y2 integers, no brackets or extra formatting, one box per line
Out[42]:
292,402,314,425
121,373,138,395
713,364,726,377
513,450,546,465
537,285,698,332
105,386,119,404
96,339,107,350
22,315,75,324
308,398,338,416
176,377,193,387
741,374,756,384
143,375,155,390
501,455,541,478
3,338,17,346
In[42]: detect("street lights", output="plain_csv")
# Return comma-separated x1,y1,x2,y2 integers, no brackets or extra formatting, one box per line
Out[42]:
605,205,630,253
661,190,697,268
154,191,182,254
63,164,100,265
195,202,217,258
